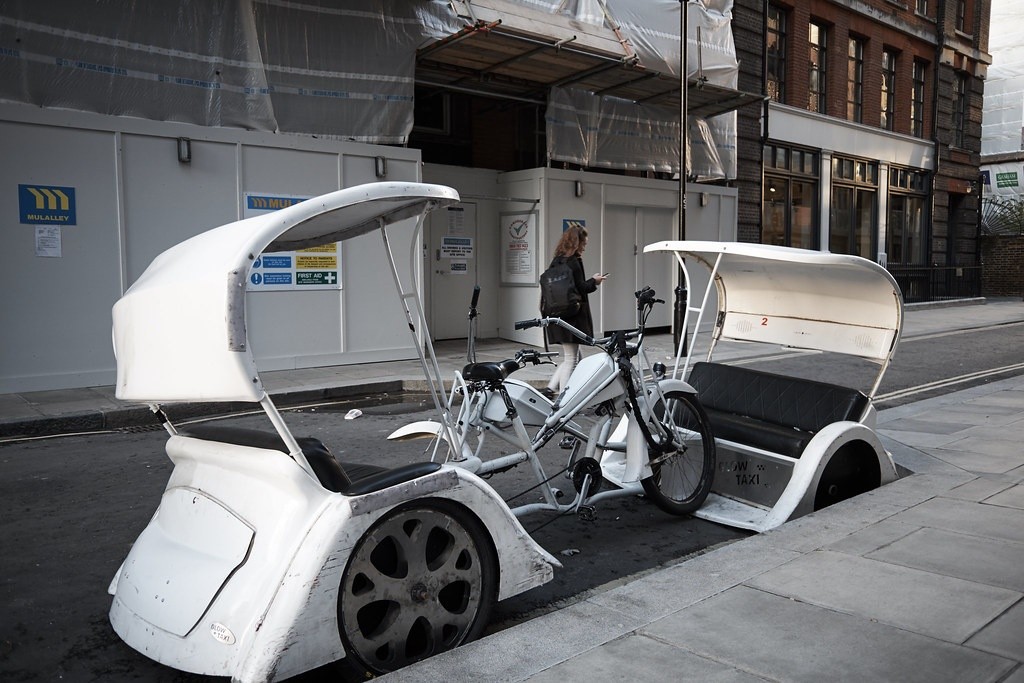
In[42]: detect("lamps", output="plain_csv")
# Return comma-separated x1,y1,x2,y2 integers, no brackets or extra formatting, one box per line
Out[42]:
574,180,585,198
374,155,389,179
700,191,710,208
176,136,192,164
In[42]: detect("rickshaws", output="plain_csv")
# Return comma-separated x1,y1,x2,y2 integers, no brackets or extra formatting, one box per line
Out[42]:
107,179,904,683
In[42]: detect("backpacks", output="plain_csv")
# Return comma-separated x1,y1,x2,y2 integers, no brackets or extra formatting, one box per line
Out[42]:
539,257,582,318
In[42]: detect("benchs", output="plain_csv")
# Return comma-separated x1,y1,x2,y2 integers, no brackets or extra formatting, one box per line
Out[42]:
179,424,443,497
649,362,871,459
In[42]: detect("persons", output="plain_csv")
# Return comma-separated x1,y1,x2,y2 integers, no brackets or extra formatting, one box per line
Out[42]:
547,225,606,396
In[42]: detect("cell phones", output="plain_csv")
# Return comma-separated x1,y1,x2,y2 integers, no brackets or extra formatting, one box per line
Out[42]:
602,273,610,277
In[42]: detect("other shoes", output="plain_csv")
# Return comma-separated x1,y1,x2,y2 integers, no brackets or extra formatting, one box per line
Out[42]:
541,387,555,400
